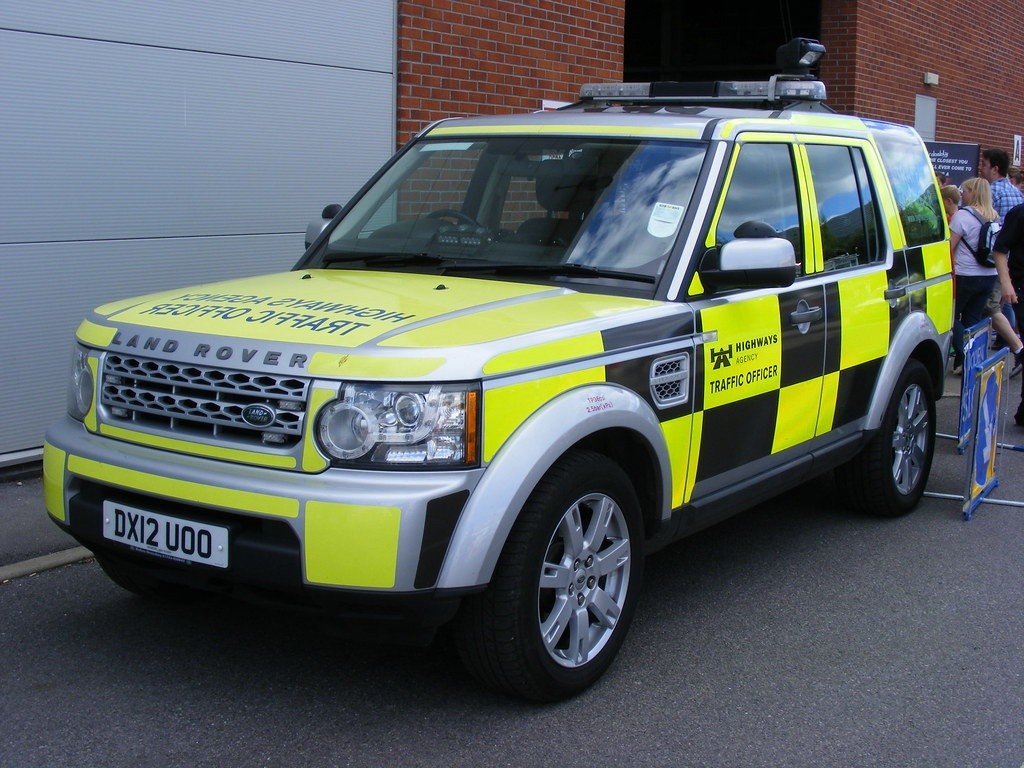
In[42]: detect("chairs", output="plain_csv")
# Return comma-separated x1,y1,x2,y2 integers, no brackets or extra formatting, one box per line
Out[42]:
514,158,594,253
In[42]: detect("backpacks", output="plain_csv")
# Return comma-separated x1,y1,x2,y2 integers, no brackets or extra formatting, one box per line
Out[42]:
955,206,1010,268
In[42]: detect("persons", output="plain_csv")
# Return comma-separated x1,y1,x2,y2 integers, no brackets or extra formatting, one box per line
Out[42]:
940,185,961,224
980,148,1024,379
992,202,1024,427
1008,166,1024,193
948,178,1001,375
935,173,946,187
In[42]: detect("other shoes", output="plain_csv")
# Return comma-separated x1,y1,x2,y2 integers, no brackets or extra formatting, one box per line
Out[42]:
1009,348,1024,378
952,353,965,374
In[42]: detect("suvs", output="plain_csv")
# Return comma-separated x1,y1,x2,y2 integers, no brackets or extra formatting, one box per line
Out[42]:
39,34,955,707
